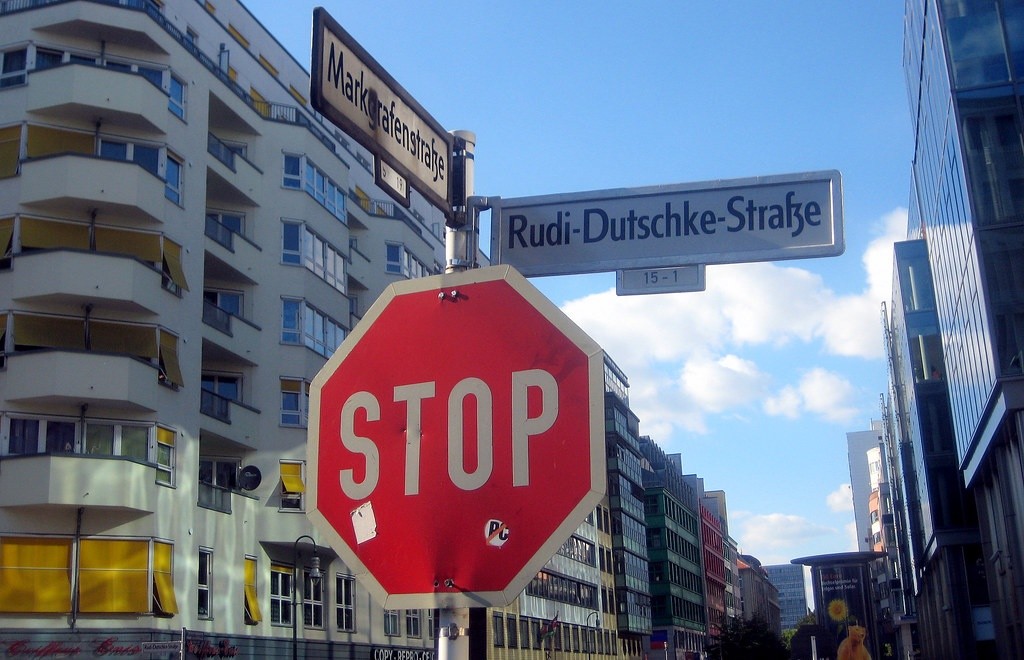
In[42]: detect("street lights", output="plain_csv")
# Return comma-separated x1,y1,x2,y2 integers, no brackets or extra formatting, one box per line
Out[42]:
587,611,600,660
293,535,322,660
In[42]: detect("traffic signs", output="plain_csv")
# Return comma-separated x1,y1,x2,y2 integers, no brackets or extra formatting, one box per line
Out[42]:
309,6,457,222
493,168,846,282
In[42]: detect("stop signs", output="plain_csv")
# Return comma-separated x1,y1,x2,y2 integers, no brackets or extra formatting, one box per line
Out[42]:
303,263,607,609
